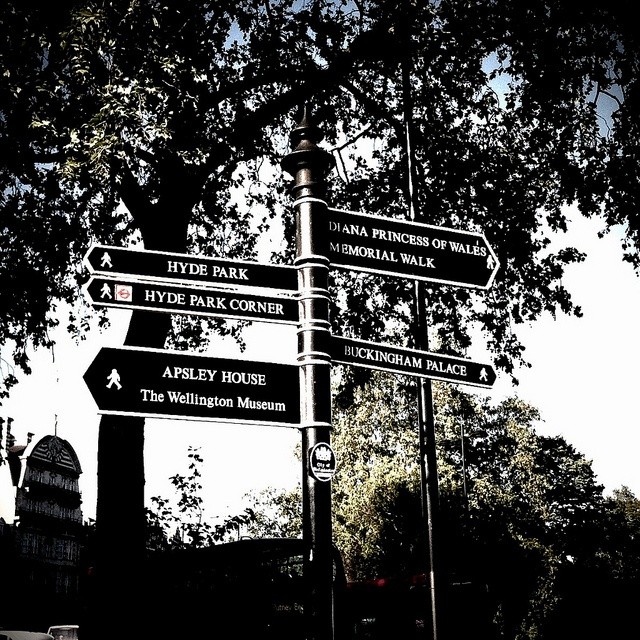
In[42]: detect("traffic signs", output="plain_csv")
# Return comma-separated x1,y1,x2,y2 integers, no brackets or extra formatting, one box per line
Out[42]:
80,275,305,325
83,346,305,428
328,208,502,291
325,335,497,389
82,243,302,294
